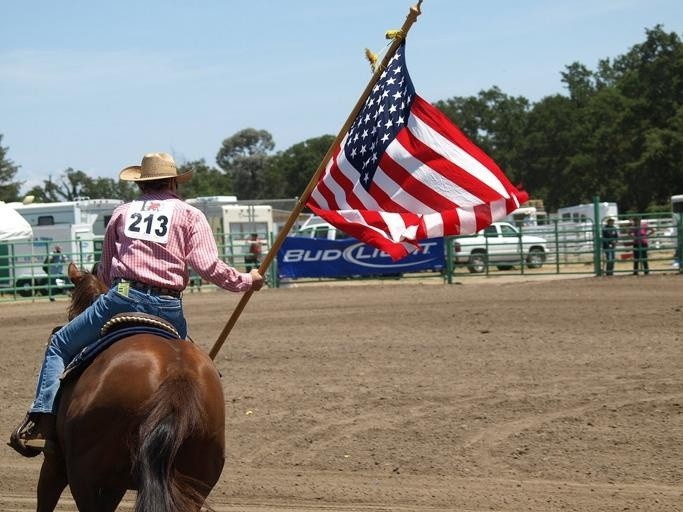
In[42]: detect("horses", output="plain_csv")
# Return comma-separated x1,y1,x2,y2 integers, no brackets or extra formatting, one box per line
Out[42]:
36,262,227,512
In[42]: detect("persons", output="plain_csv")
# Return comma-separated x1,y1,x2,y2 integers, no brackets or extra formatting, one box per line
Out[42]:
11,151,265,452
46,245,72,301
245,232,262,274
629,216,655,275
602,219,618,275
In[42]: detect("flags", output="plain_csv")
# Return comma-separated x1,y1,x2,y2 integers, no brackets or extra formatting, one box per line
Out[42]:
304,34,529,262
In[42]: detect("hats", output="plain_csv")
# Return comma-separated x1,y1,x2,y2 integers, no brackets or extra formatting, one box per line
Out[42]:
119,152,193,181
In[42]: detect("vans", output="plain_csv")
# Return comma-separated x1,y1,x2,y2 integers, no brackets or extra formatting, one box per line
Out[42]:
293,216,344,241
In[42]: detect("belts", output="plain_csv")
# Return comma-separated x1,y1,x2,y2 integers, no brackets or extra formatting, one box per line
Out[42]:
115,276,182,297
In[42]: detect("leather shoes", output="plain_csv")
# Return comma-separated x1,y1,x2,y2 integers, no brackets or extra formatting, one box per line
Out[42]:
10,413,56,450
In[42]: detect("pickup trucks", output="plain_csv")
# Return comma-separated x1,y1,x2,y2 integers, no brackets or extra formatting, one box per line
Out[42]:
442,220,551,273
